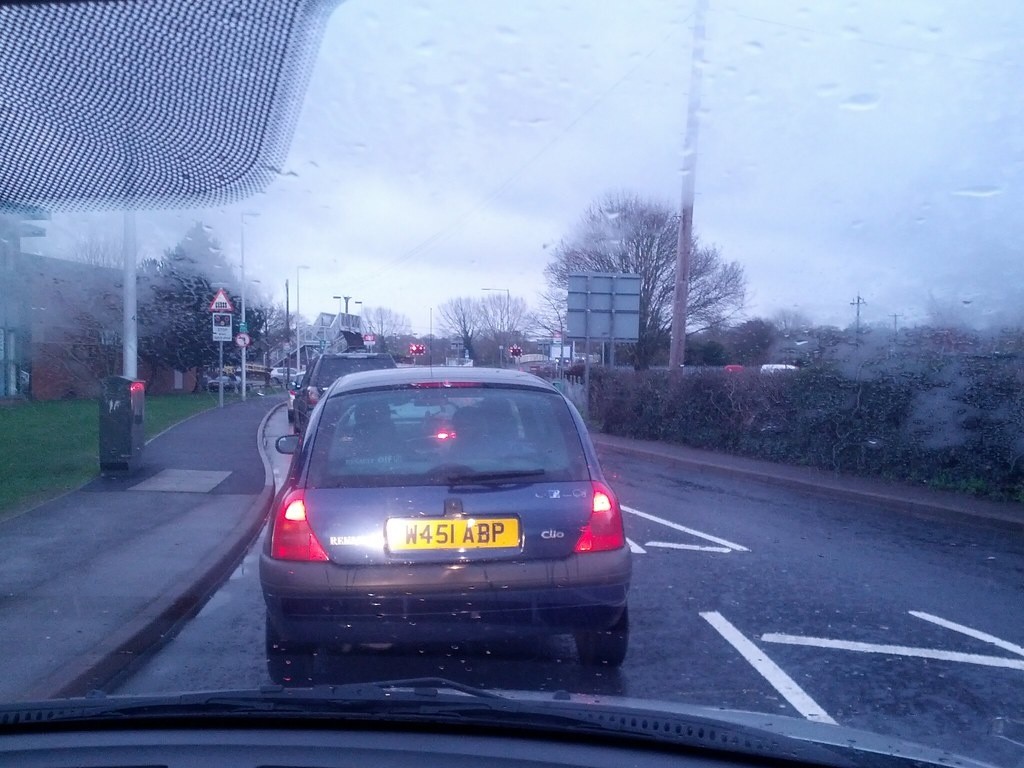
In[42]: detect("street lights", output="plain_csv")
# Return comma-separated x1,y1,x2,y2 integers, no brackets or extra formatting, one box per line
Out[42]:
296,265,310,378
239,210,262,402
333,296,342,312
482,288,510,326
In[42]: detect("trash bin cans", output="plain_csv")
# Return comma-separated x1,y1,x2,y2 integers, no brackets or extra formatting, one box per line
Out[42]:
96,374,147,480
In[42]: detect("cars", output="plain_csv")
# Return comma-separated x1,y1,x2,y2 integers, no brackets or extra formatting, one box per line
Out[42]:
256,367,633,676
267,367,297,384
209,376,251,392
287,371,306,423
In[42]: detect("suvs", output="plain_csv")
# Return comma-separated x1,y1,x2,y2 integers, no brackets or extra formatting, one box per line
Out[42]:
286,353,400,435
